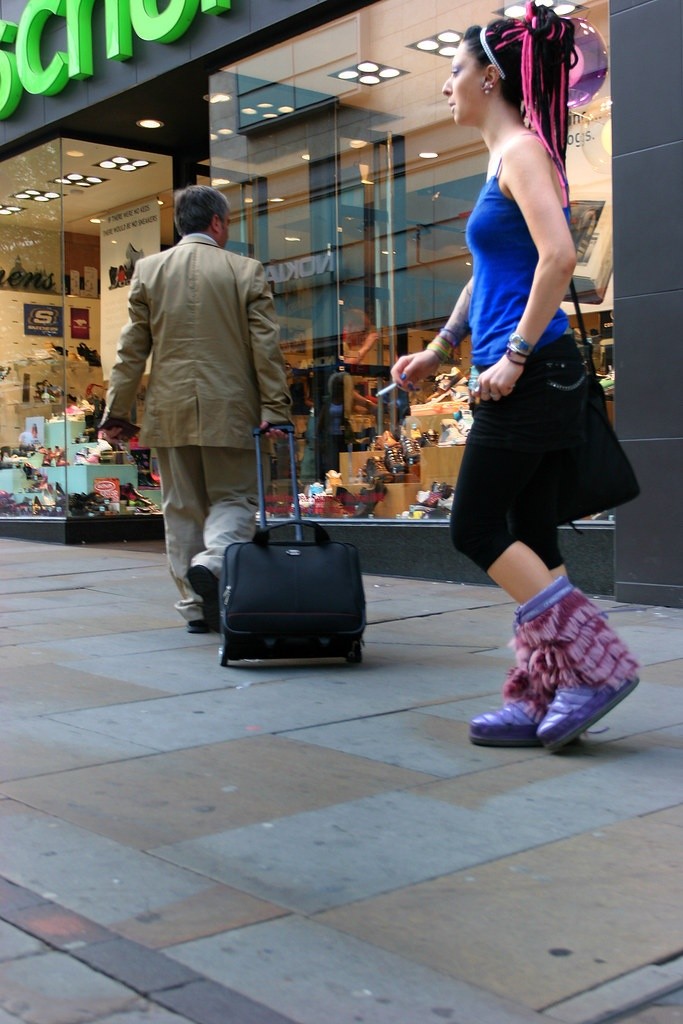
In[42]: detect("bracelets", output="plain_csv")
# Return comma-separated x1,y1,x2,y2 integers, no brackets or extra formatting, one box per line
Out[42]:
426,328,460,363
507,343,531,365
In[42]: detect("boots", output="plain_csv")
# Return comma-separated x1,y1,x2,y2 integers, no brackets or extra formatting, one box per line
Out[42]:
514,575,640,750
470,700,546,745
132,448,160,490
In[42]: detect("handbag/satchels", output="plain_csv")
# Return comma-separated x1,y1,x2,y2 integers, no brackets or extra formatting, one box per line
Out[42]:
76,342,102,368
507,360,641,533
36,380,63,399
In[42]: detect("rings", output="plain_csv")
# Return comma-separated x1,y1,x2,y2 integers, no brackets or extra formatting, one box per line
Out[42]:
490,394,498,398
471,381,480,391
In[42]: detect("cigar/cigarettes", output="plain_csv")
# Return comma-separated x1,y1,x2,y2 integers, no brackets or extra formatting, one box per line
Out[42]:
375,382,398,397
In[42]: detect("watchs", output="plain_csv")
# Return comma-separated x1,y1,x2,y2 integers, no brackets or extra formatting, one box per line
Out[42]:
509,333,529,352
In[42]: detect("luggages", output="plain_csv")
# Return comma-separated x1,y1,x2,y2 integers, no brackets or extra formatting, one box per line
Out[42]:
219,423,367,667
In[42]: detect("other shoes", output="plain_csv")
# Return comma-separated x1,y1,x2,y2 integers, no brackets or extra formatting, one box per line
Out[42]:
187,565,221,634
0,442,162,516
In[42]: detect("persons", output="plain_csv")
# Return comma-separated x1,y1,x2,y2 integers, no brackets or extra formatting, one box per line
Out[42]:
98,184,294,632
390,4,643,752
300,332,378,485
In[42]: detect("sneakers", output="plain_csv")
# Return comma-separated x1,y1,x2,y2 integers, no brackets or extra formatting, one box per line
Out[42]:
286,366,476,519
